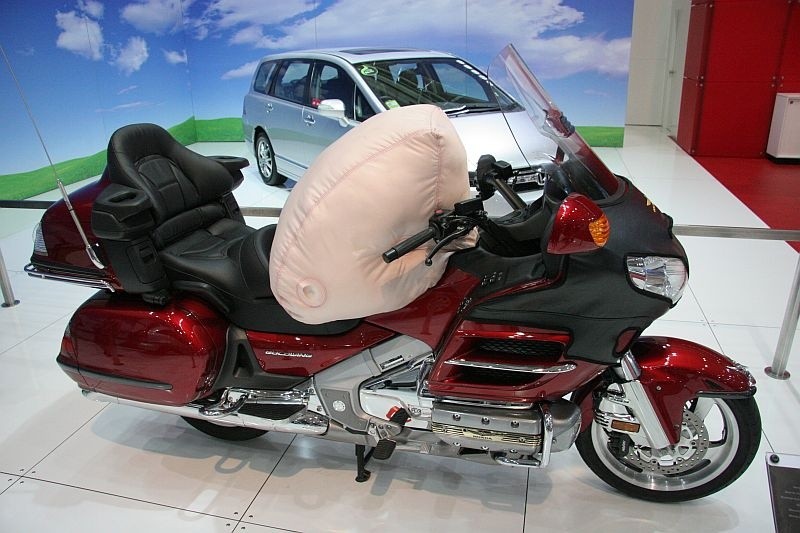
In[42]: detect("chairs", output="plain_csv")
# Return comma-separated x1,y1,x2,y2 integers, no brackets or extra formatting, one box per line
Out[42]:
398,71,419,97
98,122,255,302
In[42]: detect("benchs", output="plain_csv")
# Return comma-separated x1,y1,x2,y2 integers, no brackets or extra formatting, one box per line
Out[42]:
277,77,345,106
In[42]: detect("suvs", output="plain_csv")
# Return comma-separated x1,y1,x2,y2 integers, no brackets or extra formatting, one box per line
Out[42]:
242,46,570,218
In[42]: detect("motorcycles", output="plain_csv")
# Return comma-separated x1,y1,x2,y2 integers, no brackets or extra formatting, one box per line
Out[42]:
22,43,764,503
